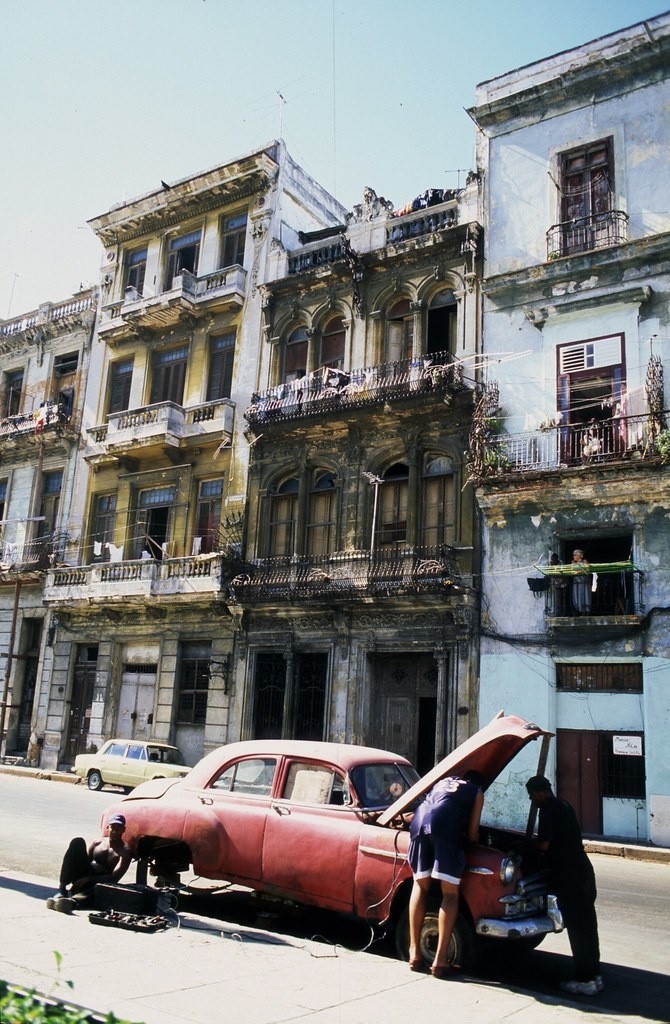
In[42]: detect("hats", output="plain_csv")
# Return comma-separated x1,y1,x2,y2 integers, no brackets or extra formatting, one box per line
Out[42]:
108,813,126,826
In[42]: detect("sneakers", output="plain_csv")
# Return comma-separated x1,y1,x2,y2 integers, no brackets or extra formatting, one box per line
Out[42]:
559,978,595,995
595,975,603,990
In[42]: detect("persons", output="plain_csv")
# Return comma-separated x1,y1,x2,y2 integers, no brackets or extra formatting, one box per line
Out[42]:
46,814,133,914
407,768,484,978
525,775,606,997
571,549,593,616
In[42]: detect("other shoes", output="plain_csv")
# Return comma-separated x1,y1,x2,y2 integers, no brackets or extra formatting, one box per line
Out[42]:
55,889,69,897
408,958,426,969
431,961,463,979
47,897,77,912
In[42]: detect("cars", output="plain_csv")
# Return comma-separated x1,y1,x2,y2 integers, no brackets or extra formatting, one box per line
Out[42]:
100,707,564,978
75,738,193,796
